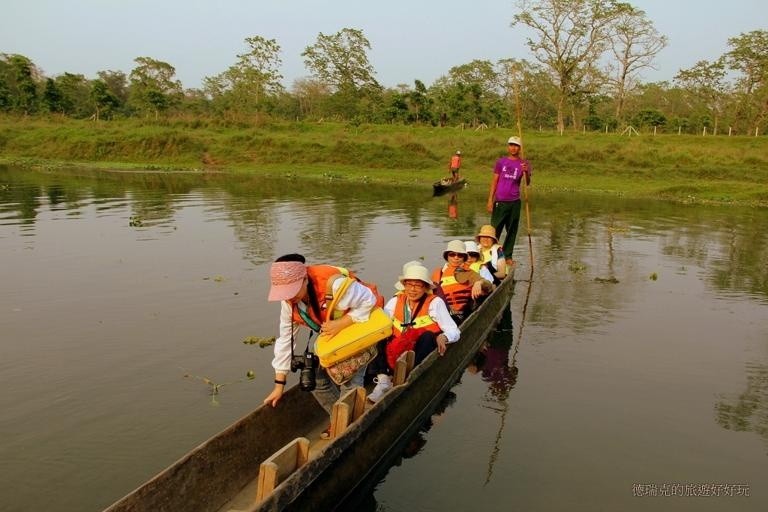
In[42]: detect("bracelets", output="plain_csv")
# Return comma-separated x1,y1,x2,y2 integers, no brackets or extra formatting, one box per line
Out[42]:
274,377,286,385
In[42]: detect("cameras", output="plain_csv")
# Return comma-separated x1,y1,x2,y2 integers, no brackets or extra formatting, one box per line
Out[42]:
291,353,318,390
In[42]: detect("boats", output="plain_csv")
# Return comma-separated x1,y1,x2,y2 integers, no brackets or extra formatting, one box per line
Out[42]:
433,186,464,199
98,262,516,511
432,177,465,192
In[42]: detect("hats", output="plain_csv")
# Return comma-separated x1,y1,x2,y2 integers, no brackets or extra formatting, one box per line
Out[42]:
268,261,307,301
476,224,498,244
508,136,521,147
393,261,437,291
464,241,480,254
442,240,468,262
456,151,461,155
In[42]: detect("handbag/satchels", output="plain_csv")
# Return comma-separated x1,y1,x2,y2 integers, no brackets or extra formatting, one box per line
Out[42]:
313,275,394,387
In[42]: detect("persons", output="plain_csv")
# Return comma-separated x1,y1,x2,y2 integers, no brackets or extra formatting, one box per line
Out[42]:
262,254,384,438
448,193,460,224
365,221,508,404
486,135,532,264
447,151,461,183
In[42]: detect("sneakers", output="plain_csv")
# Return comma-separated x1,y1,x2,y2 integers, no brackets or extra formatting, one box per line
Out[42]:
319,423,332,440
366,373,394,405
